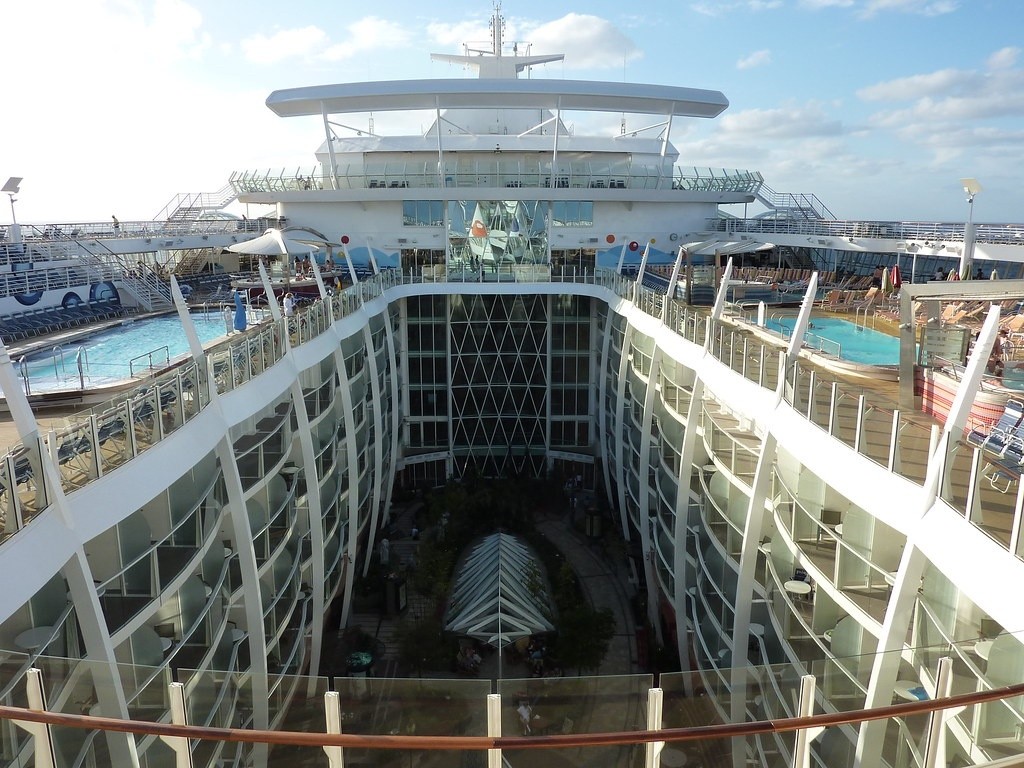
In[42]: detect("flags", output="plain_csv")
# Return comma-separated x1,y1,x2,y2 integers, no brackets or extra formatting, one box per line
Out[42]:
489,205,508,250
469,204,493,260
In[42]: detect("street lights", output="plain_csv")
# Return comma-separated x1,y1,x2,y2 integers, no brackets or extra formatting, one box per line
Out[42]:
0,175,24,225
957,175,984,281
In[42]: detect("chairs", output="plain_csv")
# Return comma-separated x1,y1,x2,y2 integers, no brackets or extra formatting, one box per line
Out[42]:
388,181,398,188
337,264,446,282
399,181,409,188
54,228,62,238
204,295,355,394
369,180,377,188
177,270,241,294
506,177,686,190
635,263,1024,493
379,181,385,188
284,462,295,479
0,295,139,345
68,229,80,238
41,229,52,237
0,363,200,537
153,623,175,651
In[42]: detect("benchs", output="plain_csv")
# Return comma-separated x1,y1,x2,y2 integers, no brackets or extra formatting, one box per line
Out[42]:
26,391,83,412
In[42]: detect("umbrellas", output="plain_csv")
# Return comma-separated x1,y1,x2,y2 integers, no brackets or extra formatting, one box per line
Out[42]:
962,261,973,281
947,268,960,281
990,268,999,280
881,265,903,300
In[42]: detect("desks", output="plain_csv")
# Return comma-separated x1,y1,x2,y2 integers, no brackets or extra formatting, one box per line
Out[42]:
160,637,172,660
66,583,106,602
688,465,994,768
231,628,244,642
297,591,306,600
15,625,60,667
282,466,300,485
89,703,101,717
205,586,212,598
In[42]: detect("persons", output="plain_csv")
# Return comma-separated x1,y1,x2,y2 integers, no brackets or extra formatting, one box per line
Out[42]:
283,293,295,316
153,261,161,272
326,260,331,272
293,255,309,269
976,268,983,280
977,330,1010,387
932,267,944,281
137,262,143,276
470,255,479,273
516,702,532,736
873,265,884,285
112,216,119,228
